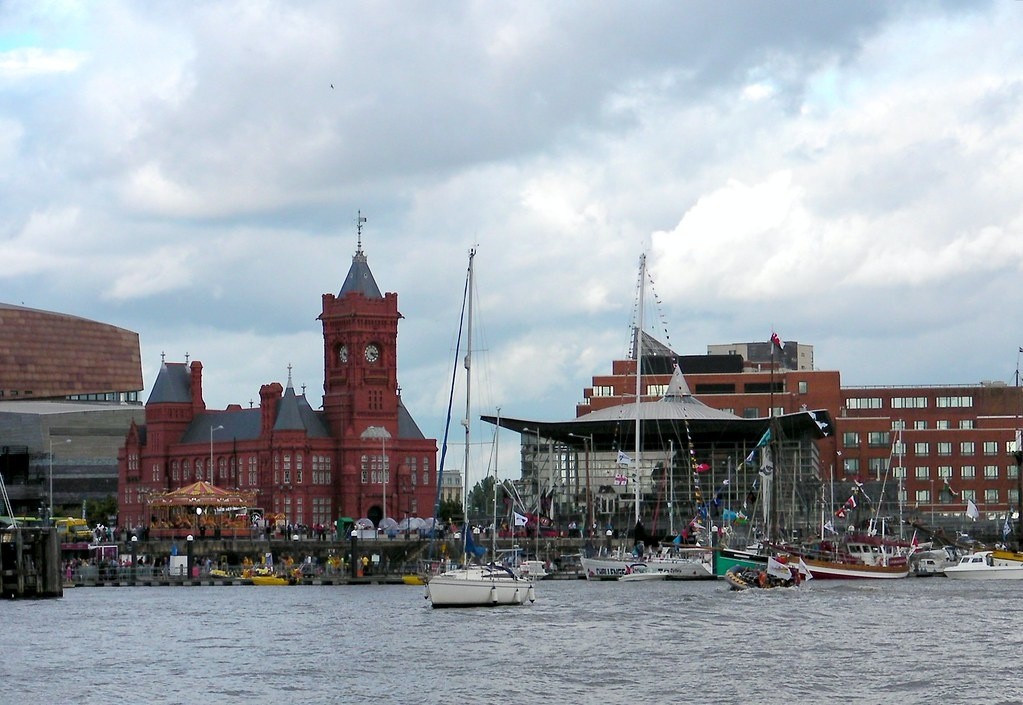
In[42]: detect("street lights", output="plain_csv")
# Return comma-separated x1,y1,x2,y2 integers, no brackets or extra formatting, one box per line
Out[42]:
277,482,294,542
368,425,388,528
400,482,415,541
48,438,71,519
137,487,149,538
219,496,224,541
190,496,197,541
210,425,224,487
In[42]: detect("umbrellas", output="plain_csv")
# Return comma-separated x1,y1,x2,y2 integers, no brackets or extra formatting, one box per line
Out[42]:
263,512,286,520
356,516,443,531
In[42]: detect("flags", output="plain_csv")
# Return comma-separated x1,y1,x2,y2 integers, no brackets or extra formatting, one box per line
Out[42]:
752,479,760,490
757,458,773,482
460,519,489,559
734,506,750,527
538,512,555,528
695,463,712,472
513,511,528,527
767,555,792,581
613,473,628,486
911,531,921,553
723,509,737,521
540,488,554,519
617,450,633,465
824,494,857,535
965,498,980,520
771,331,785,350
673,534,680,551
502,485,519,518
798,557,814,581
943,474,959,499
1019,346,1023,352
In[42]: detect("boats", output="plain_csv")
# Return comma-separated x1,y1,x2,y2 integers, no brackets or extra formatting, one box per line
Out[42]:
251,575,289,586
714,419,1023,585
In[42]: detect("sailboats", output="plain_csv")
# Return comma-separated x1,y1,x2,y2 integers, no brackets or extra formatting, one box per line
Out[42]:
422,246,550,606
563,250,715,580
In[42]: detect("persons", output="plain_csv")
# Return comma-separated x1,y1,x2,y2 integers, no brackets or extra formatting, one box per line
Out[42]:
592,522,598,535
61,555,169,582
568,520,576,537
648,541,663,562
752,524,757,538
741,564,767,588
193,551,417,577
636,541,643,562
262,520,336,542
681,525,689,544
862,514,899,536
95,524,150,543
820,540,831,561
994,542,1018,555
501,519,509,540
199,524,206,541
526,541,533,560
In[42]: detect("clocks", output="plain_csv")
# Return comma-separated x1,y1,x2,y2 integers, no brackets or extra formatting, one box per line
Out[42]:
339,345,347,363
364,344,380,362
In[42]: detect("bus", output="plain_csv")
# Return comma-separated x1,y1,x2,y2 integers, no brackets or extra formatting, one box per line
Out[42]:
11,516,93,545
